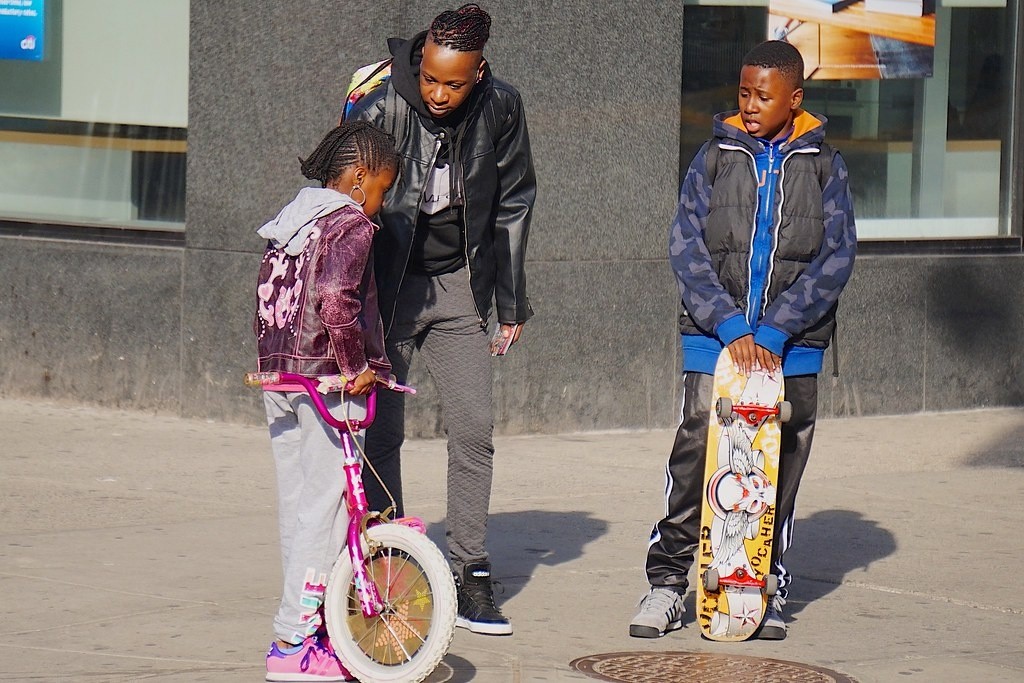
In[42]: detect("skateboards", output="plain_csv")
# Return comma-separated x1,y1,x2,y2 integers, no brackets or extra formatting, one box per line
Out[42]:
695,345,792,642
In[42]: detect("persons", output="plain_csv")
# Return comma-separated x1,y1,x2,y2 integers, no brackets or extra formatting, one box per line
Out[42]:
254,119,402,682
343,3,537,634
629,40,858,641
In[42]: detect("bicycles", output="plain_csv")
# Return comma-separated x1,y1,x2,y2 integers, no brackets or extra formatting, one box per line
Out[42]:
244,369,459,683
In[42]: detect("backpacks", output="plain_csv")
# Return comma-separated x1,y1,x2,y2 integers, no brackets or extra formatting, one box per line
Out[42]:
339,57,503,151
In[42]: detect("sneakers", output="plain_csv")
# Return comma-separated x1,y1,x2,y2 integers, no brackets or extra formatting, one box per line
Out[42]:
452,562,513,634
265,626,353,682
629,588,686,638
754,596,786,639
